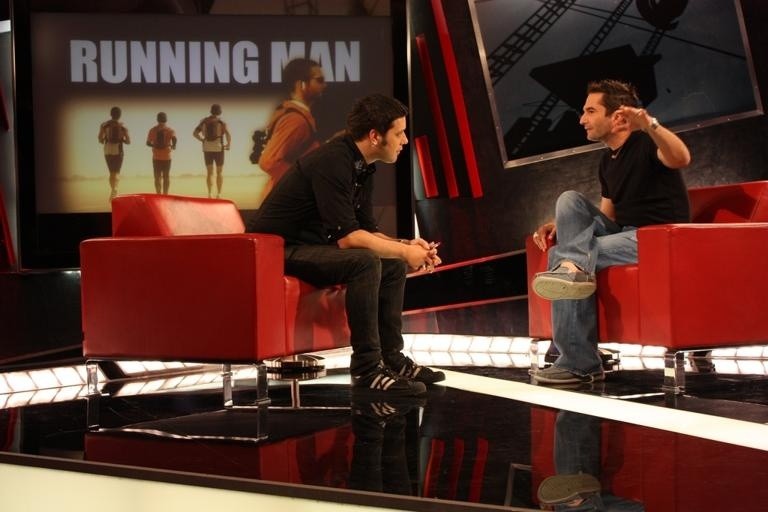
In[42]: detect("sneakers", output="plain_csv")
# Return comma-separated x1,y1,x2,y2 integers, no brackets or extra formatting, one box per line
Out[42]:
351,359,426,396
537,472,601,504
350,397,427,425
528,362,605,384
384,352,445,385
532,260,597,301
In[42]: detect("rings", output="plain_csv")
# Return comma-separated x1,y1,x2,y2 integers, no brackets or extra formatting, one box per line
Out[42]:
531,231,538,238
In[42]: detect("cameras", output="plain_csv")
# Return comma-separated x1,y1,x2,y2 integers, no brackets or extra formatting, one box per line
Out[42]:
250,130,267,164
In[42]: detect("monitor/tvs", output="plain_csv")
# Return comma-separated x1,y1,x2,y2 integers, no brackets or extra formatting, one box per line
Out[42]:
9,1,415,270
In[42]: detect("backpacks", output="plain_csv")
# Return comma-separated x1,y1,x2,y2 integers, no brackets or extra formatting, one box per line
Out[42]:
206,118,218,142
105,122,121,143
153,126,170,149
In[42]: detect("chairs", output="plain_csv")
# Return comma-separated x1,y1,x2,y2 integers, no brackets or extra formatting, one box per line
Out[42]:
78,193,353,445
525,179,768,399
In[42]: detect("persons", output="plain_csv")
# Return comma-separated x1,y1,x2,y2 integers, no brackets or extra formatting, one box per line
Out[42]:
255,57,328,207
242,90,448,398
94,105,130,205
144,111,177,196
536,410,646,512
193,102,232,200
345,402,428,499
528,77,696,382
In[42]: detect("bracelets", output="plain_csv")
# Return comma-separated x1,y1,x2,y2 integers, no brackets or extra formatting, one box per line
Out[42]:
642,116,657,136
